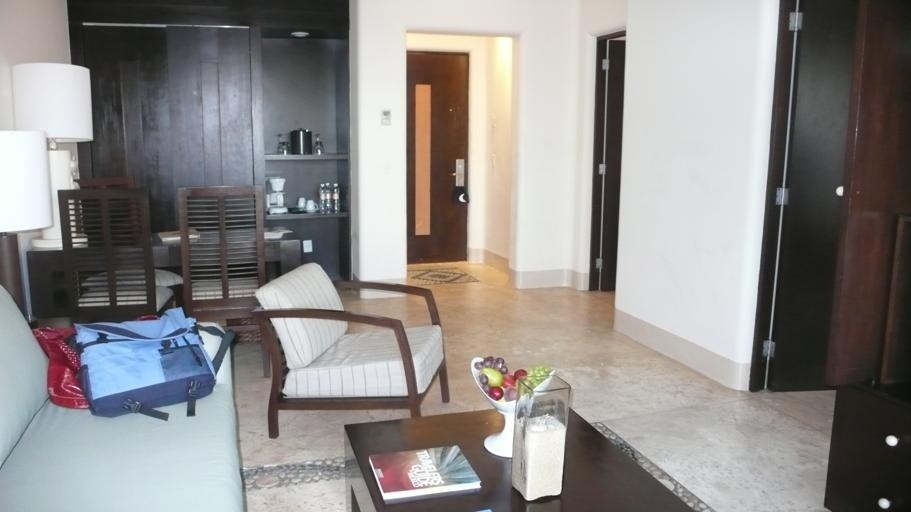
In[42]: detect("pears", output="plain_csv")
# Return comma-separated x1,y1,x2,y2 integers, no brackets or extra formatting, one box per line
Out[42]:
481,367,503,388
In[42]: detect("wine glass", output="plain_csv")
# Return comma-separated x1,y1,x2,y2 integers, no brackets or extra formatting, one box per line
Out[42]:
470,357,556,459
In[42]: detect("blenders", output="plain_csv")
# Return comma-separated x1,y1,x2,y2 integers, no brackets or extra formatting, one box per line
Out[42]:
264,175,288,214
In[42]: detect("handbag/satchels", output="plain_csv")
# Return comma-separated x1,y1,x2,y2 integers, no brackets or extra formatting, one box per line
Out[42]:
72,306,240,422
32,312,162,410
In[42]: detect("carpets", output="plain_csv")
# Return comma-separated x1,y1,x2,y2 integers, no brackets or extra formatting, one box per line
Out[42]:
242,422,716,512
408,267,480,286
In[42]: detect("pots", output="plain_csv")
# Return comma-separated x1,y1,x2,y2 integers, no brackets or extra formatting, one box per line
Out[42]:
290,128,312,154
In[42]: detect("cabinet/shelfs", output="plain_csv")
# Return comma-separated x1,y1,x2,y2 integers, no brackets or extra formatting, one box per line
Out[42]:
68,1,350,307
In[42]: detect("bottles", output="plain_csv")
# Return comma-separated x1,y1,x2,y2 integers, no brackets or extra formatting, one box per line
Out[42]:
318,182,340,212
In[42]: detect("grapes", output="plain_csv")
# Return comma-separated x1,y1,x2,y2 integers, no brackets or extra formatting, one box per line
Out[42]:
519,366,550,393
483,385,489,393
480,374,490,384
474,356,508,374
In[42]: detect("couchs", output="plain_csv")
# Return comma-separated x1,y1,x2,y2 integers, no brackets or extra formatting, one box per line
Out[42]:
0,283,244,512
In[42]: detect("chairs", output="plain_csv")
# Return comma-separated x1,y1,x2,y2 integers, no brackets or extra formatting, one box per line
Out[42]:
74,175,139,246
58,190,177,326
176,185,274,377
251,262,450,438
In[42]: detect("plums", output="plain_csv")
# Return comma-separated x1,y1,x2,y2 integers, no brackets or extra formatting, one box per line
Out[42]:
490,387,503,400
503,374,515,388
505,389,518,401
514,369,527,381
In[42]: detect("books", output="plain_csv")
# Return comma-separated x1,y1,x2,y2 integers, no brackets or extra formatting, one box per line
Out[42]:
263,223,296,240
159,227,202,242
368,443,485,503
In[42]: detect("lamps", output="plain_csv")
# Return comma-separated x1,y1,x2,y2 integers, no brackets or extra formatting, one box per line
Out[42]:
12,62,94,247
0,129,54,329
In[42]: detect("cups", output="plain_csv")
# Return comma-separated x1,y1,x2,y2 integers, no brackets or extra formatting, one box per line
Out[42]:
298,197,318,211
512,374,570,501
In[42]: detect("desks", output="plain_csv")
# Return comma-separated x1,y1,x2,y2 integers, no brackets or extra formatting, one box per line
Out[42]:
344,398,698,512
26,228,302,318
